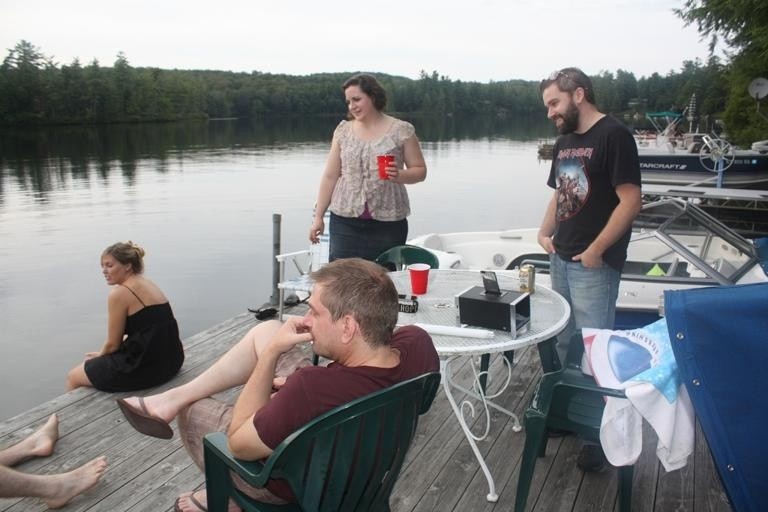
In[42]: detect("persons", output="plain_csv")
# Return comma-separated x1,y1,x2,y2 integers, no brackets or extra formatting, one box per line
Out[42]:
0,411,107,509
114,257,439,512
536,67,643,476
65,240,185,391
310,72,428,270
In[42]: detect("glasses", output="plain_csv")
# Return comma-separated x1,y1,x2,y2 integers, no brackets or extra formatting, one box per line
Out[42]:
546,68,573,82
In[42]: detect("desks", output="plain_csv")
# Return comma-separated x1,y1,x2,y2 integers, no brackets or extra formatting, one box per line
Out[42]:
387,268,573,503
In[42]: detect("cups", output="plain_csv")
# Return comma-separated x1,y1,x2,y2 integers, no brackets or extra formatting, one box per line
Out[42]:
407,263,431,296
376,154,395,179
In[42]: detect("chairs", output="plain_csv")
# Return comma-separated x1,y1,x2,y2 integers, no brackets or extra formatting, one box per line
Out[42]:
375,244,461,268
201,371,440,512
512,315,681,511
275,247,330,320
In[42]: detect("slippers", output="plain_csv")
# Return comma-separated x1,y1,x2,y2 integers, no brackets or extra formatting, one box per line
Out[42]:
116,396,174,440
174,490,207,512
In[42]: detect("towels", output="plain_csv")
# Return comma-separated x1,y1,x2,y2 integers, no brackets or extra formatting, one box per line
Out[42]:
579,313,698,473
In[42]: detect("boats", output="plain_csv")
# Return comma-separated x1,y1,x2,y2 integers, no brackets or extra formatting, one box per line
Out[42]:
382,185,768,330
624,91,768,173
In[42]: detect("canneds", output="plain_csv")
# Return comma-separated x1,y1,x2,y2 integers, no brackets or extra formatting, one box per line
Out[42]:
519,264,535,295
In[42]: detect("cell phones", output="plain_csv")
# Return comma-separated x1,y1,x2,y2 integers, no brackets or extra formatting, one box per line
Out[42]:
480,271,501,294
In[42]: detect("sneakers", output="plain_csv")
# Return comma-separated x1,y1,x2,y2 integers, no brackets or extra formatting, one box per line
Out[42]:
545,426,572,437
576,444,609,474
